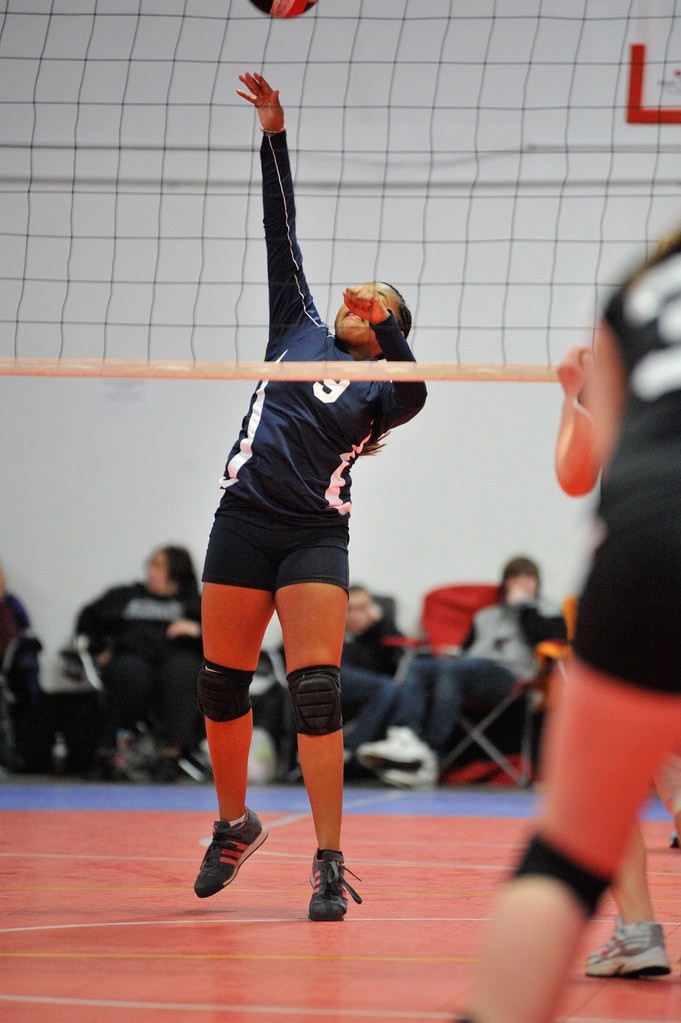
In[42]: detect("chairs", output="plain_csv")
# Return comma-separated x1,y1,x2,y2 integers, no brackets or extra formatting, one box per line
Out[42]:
0,583,586,784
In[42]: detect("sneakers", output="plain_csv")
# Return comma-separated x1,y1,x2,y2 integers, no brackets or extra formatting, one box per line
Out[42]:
377,744,439,789
193,804,269,899
306,846,348,920
585,921,670,978
355,726,424,772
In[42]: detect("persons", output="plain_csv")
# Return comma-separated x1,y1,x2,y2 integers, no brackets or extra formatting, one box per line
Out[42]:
352,552,568,783
72,544,214,783
581,737,681,984
192,70,430,921
459,228,681,1018
257,581,399,787
